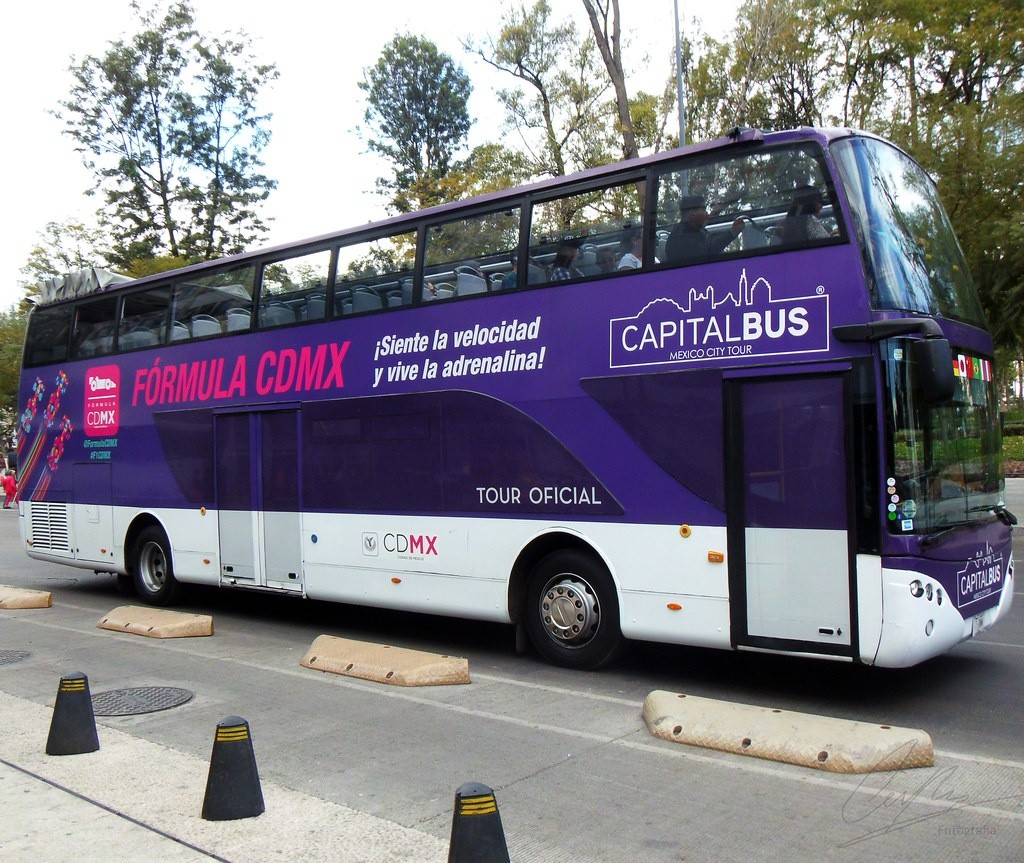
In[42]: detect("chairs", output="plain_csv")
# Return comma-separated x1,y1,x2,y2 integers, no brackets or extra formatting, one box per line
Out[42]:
82,215,778,358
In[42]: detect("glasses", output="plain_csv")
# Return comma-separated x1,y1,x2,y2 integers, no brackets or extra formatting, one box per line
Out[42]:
599,255,615,264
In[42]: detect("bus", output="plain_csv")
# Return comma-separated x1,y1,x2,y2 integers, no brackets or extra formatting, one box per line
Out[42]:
14,125,1021,673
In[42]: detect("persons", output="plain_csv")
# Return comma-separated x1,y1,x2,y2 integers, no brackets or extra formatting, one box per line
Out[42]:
617,225,660,271
1,470,18,509
664,196,745,260
551,236,586,281
502,248,518,289
596,247,617,273
774,185,831,244
453,261,484,297
